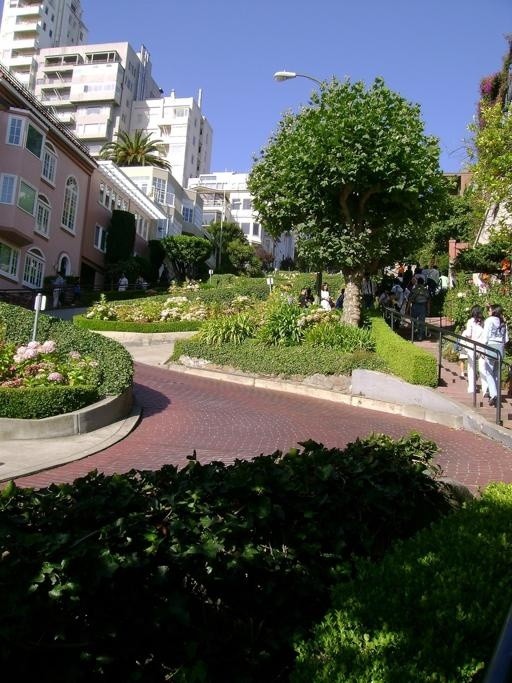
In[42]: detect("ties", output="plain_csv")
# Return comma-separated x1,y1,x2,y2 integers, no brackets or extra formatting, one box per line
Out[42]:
489,396,498,406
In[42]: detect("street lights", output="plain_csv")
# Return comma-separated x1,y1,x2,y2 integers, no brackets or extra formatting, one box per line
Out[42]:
219,200,241,274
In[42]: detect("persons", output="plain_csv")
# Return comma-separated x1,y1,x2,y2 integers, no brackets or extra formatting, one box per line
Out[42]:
455,325,467,380
297,282,345,311
135,274,143,284
362,261,448,340
464,304,489,398
117,272,129,292
52,272,64,308
476,304,509,406
